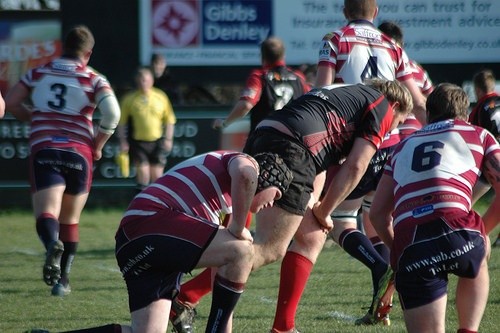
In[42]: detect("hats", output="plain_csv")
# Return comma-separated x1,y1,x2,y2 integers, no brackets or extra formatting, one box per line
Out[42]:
253,152,293,195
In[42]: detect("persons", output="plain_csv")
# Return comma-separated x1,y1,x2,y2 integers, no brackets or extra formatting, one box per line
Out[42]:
170,77,414,333
468,71,500,250
315,1,428,326
6,25,121,297
0,91,6,119
377,22,435,140
120,69,176,192
21,150,293,333
151,54,175,106
214,38,311,228
369,82,500,333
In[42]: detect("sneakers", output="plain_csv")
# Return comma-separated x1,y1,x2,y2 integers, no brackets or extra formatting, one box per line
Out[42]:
49,281,72,296
170,298,197,333
373,264,397,320
42,240,64,286
353,312,390,327
